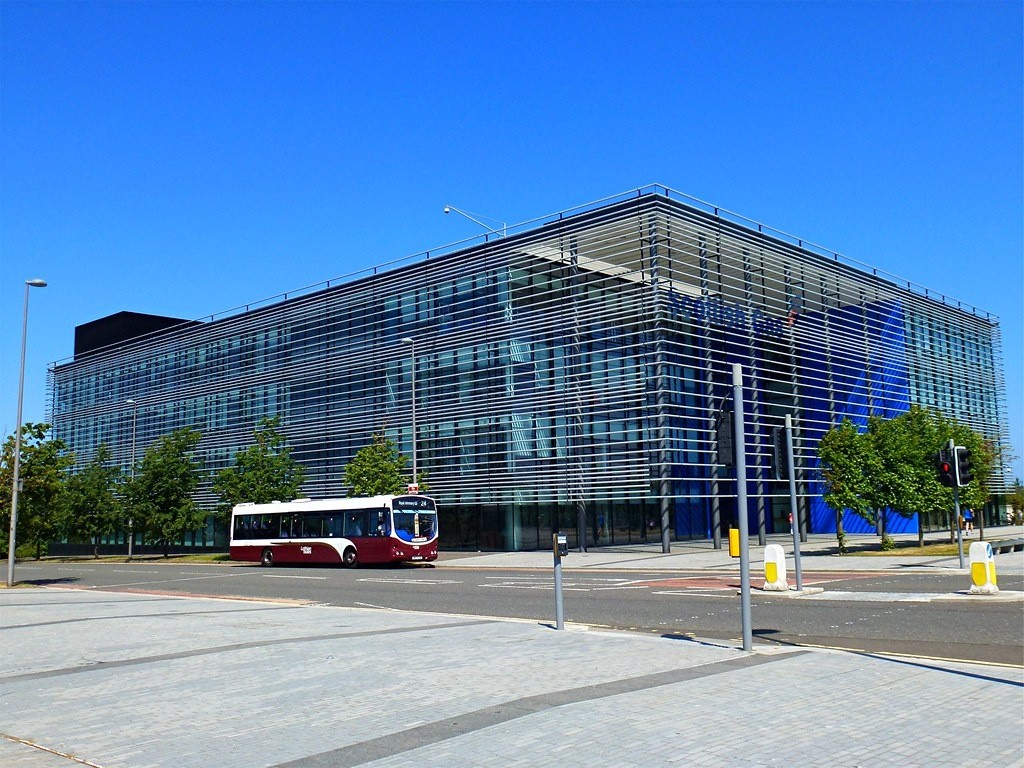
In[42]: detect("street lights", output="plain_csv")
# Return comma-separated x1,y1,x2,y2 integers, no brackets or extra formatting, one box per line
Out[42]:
7,278,48,584
402,337,416,484
126,399,137,560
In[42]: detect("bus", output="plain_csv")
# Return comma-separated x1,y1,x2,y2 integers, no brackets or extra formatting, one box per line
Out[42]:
229,493,439,570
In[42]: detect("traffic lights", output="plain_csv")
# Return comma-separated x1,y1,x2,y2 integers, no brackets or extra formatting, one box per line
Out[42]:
954,445,974,488
935,448,956,486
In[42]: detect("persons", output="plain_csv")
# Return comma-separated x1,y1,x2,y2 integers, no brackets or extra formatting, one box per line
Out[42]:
963,506,975,536
992,512,1022,526
